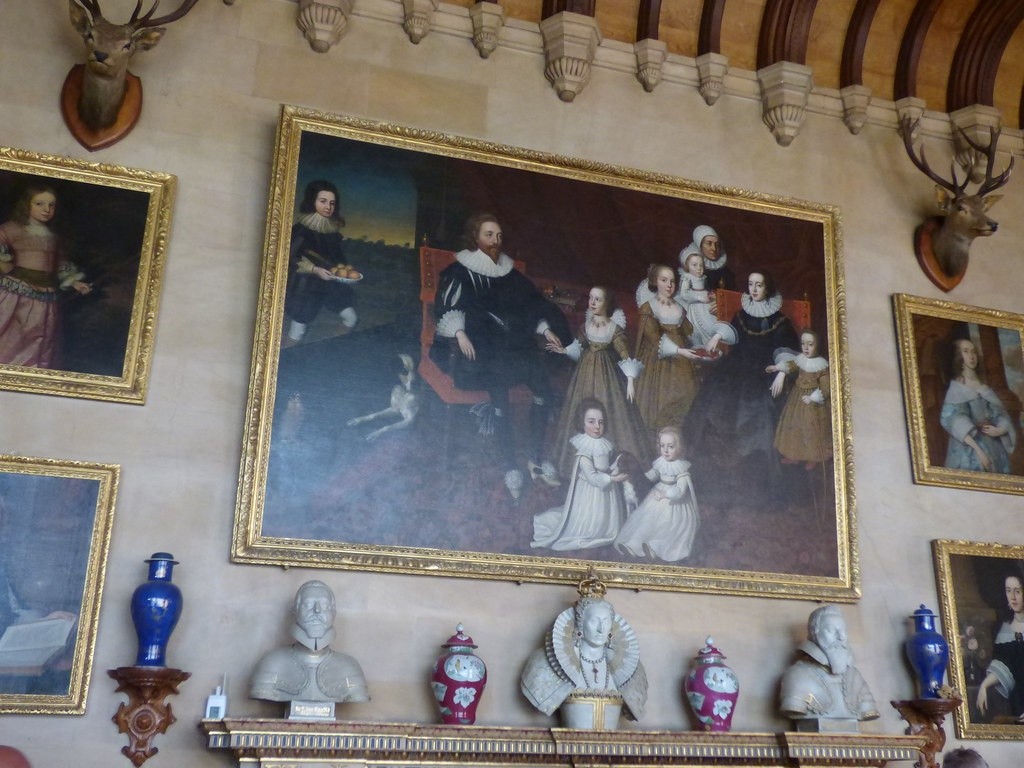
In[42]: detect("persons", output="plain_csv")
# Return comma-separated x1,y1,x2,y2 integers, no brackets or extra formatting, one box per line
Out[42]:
251,582,368,699
545,563,640,694
778,600,880,720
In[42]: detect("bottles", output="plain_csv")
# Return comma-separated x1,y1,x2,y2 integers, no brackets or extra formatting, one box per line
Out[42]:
431,621,488,724
206,687,227,720
906,604,949,698
684,635,739,733
131,552,183,669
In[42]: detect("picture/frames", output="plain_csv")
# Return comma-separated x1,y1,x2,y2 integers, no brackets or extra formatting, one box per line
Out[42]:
892,294,1023,494
1,455,123,718
228,104,860,605
0,146,178,403
933,537,1024,742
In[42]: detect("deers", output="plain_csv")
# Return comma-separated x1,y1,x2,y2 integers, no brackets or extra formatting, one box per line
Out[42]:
902,113,1015,278
67,1,200,134
